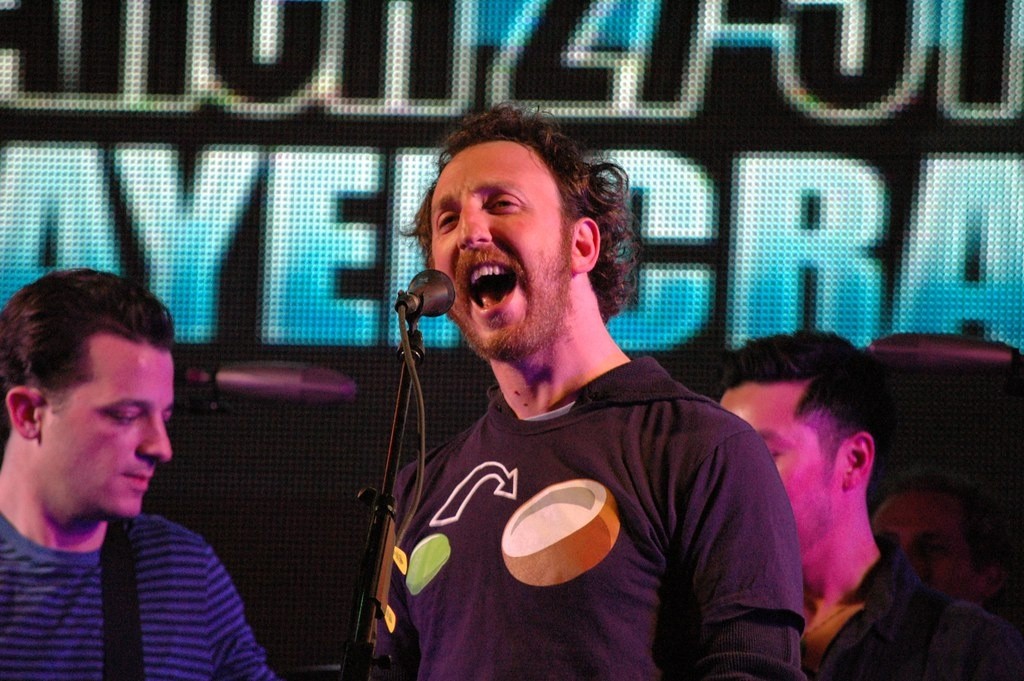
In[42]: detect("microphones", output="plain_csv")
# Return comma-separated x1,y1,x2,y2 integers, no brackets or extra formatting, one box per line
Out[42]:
173,360,357,404
394,270,455,317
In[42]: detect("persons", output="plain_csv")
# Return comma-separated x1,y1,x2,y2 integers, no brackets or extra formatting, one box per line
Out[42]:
714,329,1023,681
0,269,278,681
869,473,1008,615
356,108,808,679
